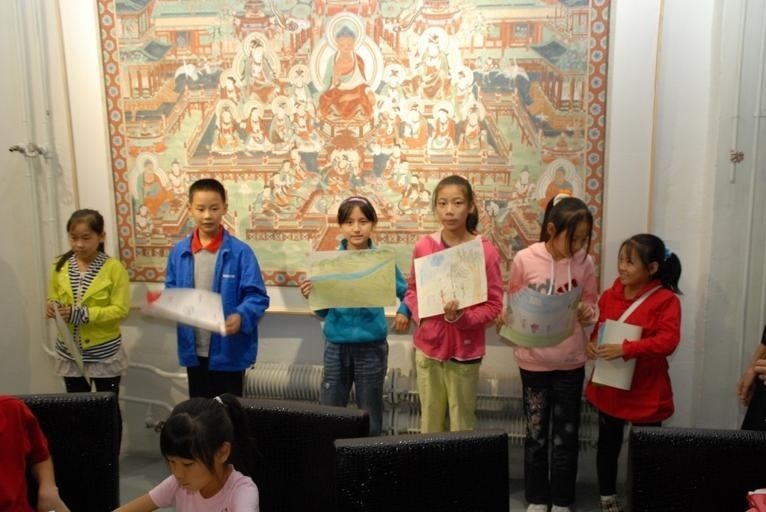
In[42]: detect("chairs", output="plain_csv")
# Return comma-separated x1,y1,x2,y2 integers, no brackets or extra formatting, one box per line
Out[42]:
527,504,570,512
602,494,622,512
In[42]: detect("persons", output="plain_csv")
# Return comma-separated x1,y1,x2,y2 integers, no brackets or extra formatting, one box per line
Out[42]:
165,179,270,398
585,234,683,512
736,323,766,430
404,176,504,435
299,197,411,437
46,209,130,395
112,392,261,512
494,193,600,512
0,395,71,512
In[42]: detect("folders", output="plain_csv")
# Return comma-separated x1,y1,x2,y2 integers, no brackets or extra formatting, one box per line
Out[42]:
146,365,631,451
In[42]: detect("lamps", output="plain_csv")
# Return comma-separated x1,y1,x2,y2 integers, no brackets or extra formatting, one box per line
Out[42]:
1,391,121,512
333,430,510,512
628,427,765,512
227,398,368,511
